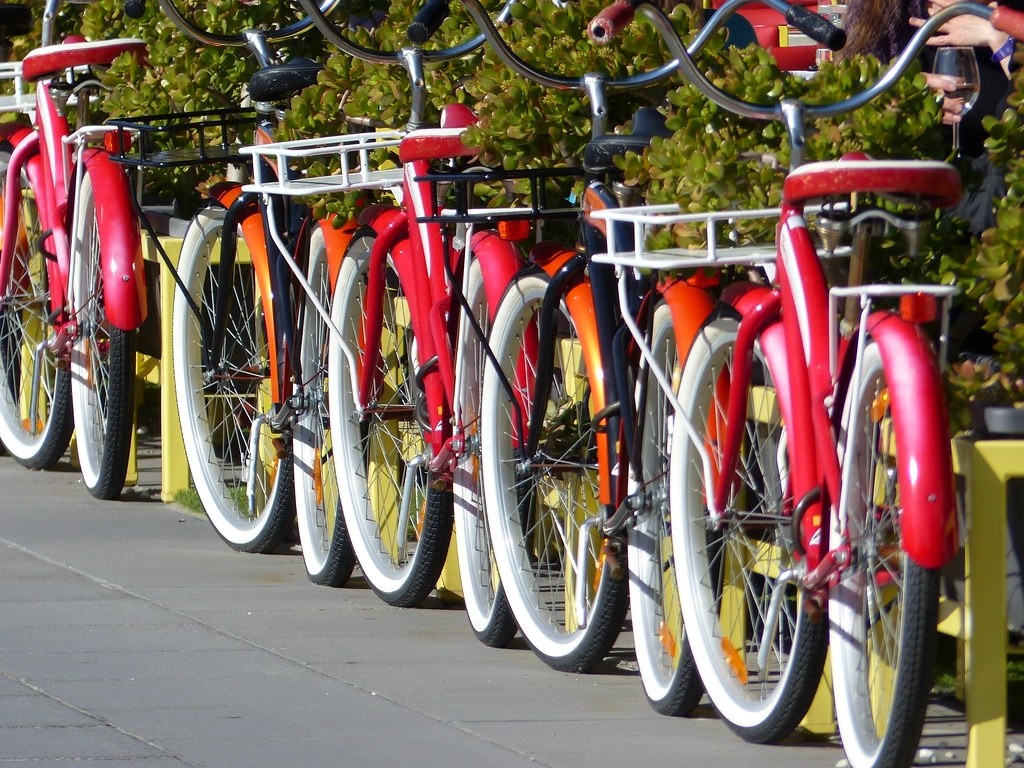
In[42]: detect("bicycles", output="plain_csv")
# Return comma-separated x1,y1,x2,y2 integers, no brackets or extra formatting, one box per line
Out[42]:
0,1,1023,768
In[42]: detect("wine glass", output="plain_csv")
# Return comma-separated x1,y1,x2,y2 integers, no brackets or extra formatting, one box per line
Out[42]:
933,46,981,164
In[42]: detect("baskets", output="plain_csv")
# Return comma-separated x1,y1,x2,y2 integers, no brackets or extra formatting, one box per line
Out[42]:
1,62,98,110
107,104,259,168
591,203,852,268
238,129,406,197
412,168,589,223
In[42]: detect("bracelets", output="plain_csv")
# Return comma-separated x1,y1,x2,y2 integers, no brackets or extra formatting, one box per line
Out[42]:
991,35,1016,65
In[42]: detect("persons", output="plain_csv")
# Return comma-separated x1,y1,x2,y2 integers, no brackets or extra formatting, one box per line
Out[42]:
834,0,1024,242
